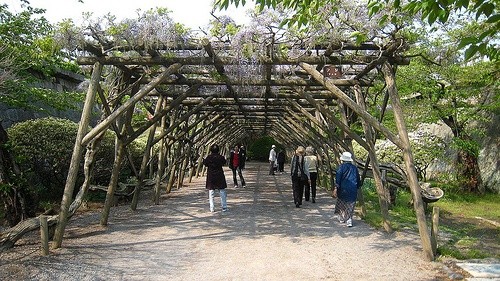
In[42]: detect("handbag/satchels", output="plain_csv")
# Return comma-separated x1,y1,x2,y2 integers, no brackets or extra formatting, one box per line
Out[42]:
301,171,308,184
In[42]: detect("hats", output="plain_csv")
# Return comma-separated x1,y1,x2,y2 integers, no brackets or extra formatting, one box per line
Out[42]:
272,145,276,149
340,152,353,162
296,147,306,156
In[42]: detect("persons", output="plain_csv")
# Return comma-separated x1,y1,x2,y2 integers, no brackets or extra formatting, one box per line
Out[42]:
277,148,285,173
229,145,246,188
333,152,359,227
304,147,318,203
290,146,306,207
239,144,246,170
204,144,230,212
269,145,276,175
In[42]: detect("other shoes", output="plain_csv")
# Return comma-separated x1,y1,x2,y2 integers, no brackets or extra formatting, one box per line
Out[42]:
296,202,299,208
234,186,238,188
346,219,353,227
222,208,230,212
340,219,344,224
243,186,246,188
211,209,215,213
312,197,315,204
